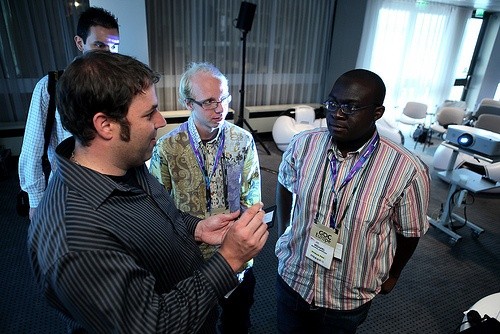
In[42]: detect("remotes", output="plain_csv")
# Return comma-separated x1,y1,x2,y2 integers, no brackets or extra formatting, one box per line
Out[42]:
482,175,497,184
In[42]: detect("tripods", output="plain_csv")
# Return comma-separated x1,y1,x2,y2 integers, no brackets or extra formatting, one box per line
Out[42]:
236,30,270,155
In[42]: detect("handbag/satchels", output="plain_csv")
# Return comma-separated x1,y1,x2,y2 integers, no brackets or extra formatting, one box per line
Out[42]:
412,123,429,153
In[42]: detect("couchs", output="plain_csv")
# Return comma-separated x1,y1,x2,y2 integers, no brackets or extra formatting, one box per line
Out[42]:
272,102,405,151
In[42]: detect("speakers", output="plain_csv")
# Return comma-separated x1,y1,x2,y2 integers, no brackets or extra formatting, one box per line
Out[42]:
235,1,256,31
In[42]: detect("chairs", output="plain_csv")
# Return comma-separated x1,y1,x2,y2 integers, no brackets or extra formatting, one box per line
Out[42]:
397,98,500,153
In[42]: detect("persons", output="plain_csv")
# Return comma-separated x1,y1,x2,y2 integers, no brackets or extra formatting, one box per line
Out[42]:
19,6,122,220
27,49,269,334
275,69,430,334
149,63,261,334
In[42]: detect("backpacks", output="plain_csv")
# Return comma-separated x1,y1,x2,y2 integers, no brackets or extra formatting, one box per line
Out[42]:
15,70,64,217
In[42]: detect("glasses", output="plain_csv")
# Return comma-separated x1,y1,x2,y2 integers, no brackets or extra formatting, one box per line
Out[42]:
187,93,232,110
322,99,375,114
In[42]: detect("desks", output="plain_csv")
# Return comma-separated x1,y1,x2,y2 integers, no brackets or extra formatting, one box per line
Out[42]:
425,138,500,247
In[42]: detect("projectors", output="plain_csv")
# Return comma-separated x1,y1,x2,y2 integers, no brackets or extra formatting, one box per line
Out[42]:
445,124,500,157
